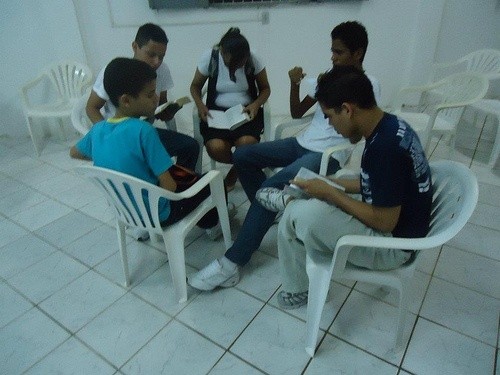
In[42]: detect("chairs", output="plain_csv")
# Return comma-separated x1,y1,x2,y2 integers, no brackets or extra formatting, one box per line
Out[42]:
19,49,500,357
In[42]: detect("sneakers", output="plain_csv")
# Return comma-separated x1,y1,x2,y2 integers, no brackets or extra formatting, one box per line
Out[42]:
187,257,240,291
276,290,330,308
256,187,287,212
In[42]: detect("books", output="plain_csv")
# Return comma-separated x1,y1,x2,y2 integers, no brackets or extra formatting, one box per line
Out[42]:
206,105,251,131
284,167,345,199
139,96,192,121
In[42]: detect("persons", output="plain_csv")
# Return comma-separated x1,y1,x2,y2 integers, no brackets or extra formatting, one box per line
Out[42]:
256,65,433,310
86,23,201,170
70,56,238,242
190,27,272,192
186,22,381,291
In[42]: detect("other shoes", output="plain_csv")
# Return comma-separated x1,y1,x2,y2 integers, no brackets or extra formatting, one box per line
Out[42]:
135,230,148,241
224,182,234,192
207,202,236,239
